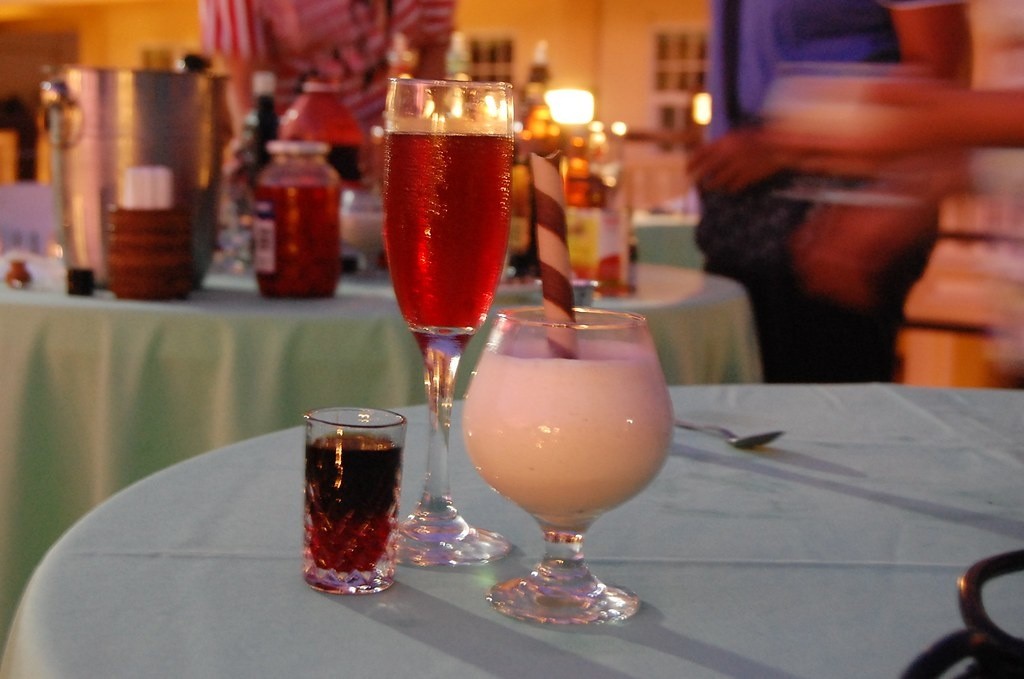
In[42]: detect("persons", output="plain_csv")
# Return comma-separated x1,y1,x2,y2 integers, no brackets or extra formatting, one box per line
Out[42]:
198,0,455,191
685,0,1024,384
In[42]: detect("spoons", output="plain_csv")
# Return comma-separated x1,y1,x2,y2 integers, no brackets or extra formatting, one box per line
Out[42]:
674,420,783,448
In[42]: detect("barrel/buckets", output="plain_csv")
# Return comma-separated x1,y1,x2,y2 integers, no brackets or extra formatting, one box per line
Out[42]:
37,64,213,289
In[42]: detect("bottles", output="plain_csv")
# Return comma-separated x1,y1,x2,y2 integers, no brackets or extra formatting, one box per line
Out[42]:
245,72,280,186
107,167,192,301
277,71,362,189
509,38,631,305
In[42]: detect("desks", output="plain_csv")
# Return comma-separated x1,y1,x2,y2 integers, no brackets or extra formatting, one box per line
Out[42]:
0,262,762,645
635,208,703,269
0,385,1024,679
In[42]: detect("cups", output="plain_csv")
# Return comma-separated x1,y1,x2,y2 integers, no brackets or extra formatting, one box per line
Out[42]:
302,406,408,595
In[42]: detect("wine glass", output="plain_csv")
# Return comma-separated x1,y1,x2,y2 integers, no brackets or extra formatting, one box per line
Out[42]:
341,185,389,284
461,307,673,626
381,78,515,571
